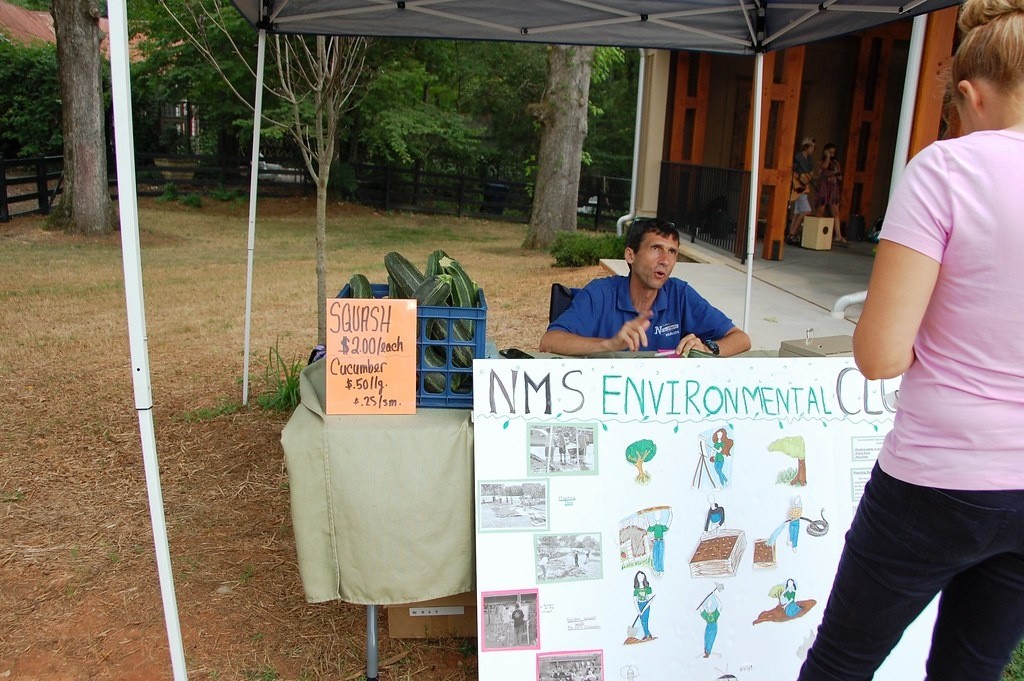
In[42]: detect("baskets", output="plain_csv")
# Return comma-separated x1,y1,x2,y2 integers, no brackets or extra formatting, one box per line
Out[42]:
336,282,487,409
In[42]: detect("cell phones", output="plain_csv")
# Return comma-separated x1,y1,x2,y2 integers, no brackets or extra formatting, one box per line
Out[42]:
499,348,534,359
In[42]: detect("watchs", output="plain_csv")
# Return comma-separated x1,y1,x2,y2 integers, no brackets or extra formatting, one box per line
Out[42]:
702,337,721,356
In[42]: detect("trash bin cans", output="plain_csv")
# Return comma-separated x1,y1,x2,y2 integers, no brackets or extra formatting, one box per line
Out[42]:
483,183,509,214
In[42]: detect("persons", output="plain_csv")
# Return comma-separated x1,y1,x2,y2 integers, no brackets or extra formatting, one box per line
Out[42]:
544,427,557,466
548,657,601,681
555,428,567,465
573,551,580,568
575,428,587,464
484,597,525,644
785,134,818,248
537,217,752,359
538,552,552,580
782,0,1024,681
816,140,851,249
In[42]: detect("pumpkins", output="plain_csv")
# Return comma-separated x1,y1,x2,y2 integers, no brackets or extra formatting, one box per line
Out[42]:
348,248,477,394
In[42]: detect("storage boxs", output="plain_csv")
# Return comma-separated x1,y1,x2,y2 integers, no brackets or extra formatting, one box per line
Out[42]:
335,282,488,408
779,335,853,357
385,591,477,639
802,215,834,249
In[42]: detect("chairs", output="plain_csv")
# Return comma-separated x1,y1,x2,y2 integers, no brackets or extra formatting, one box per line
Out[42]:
550,283,573,322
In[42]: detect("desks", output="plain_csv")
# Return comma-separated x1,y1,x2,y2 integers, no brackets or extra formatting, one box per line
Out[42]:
281,347,780,681
